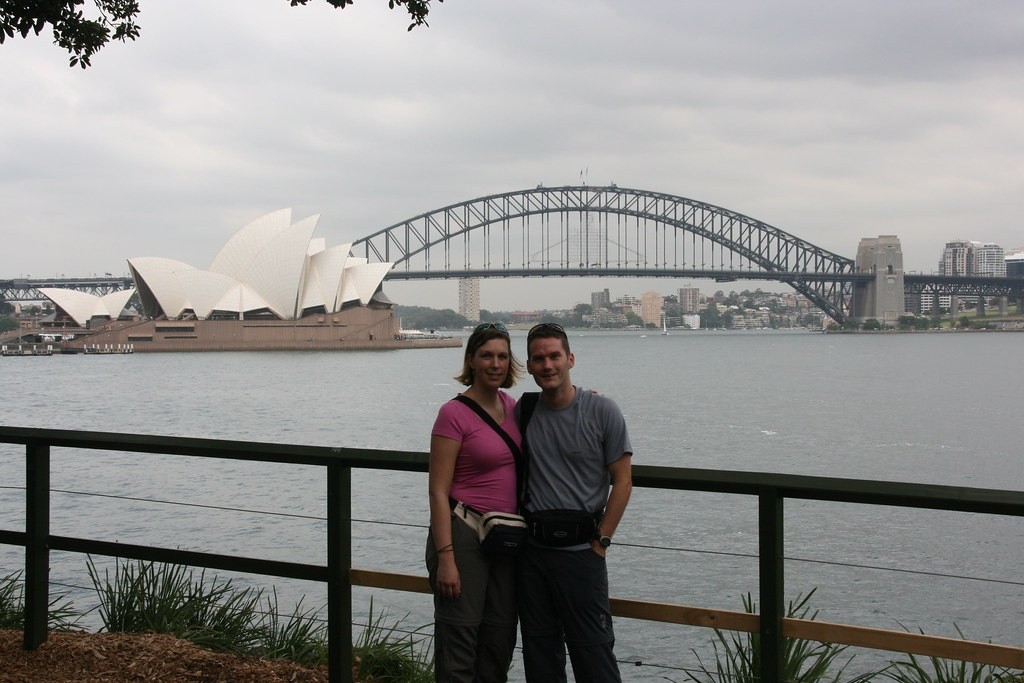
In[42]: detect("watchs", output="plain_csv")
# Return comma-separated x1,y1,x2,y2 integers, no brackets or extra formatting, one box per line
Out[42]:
595,529,611,547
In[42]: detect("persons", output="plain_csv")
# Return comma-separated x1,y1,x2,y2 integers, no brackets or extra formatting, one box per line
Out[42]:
425,322,603,683
518,324,632,683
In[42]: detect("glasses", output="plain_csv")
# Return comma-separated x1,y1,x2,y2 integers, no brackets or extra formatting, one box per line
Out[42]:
528,323,564,337
473,323,508,335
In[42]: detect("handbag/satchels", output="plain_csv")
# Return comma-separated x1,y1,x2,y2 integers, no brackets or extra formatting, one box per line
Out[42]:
520,504,602,546
451,498,526,556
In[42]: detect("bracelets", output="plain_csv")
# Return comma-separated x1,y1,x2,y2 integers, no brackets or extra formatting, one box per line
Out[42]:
437,544,455,552
